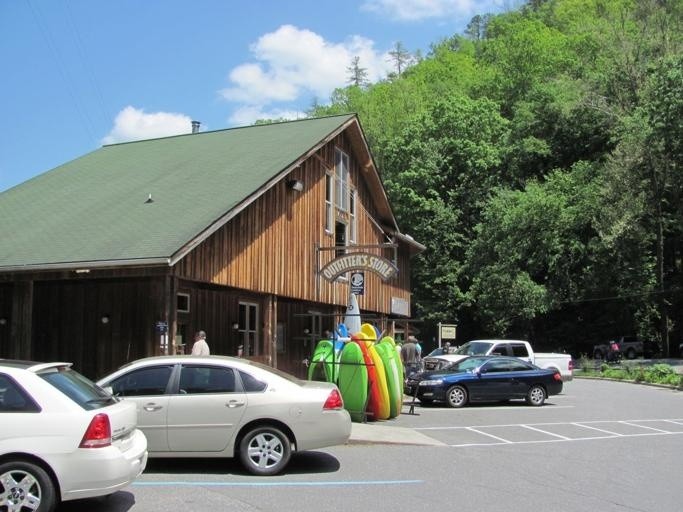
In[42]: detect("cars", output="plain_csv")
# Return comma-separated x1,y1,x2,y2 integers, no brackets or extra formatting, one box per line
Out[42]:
405,354,564,409
0,356,150,511
95,354,352,476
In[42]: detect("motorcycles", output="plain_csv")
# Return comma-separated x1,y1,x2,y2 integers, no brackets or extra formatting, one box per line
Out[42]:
602,351,622,365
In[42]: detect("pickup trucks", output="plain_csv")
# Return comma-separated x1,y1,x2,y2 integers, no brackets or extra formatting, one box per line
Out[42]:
593,335,644,359
421,340,574,394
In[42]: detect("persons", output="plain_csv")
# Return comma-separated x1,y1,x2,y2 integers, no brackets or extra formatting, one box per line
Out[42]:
603,340,619,363
395,335,461,379
190,330,211,385
